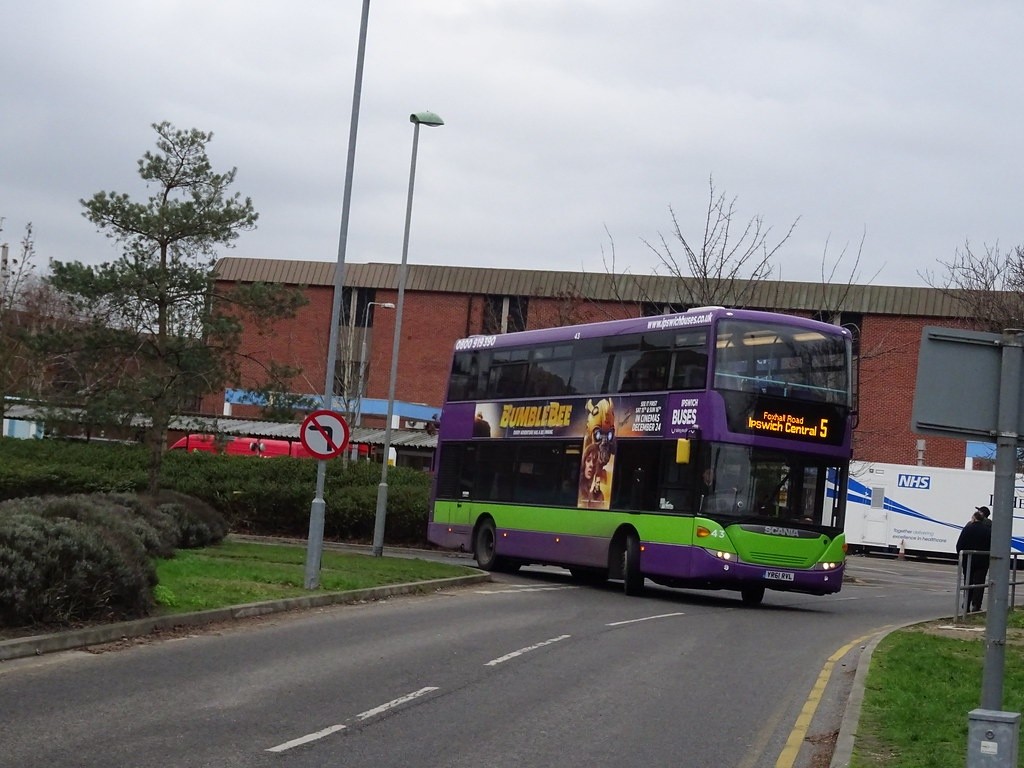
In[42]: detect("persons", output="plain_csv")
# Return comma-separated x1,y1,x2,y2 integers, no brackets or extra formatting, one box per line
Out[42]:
956,506,992,612
580,443,604,507
703,469,737,495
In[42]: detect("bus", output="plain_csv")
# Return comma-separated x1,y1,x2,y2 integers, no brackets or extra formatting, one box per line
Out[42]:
170,434,371,464
426,305,863,601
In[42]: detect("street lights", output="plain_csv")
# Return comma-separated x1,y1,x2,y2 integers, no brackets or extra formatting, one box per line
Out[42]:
353,301,396,428
380,111,445,492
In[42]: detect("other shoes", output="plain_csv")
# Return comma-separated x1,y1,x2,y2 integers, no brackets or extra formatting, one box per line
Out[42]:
972,606,983,612
962,604,970,612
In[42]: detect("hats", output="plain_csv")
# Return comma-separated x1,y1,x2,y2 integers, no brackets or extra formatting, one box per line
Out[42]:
580,436,597,466
975,506,990,517
